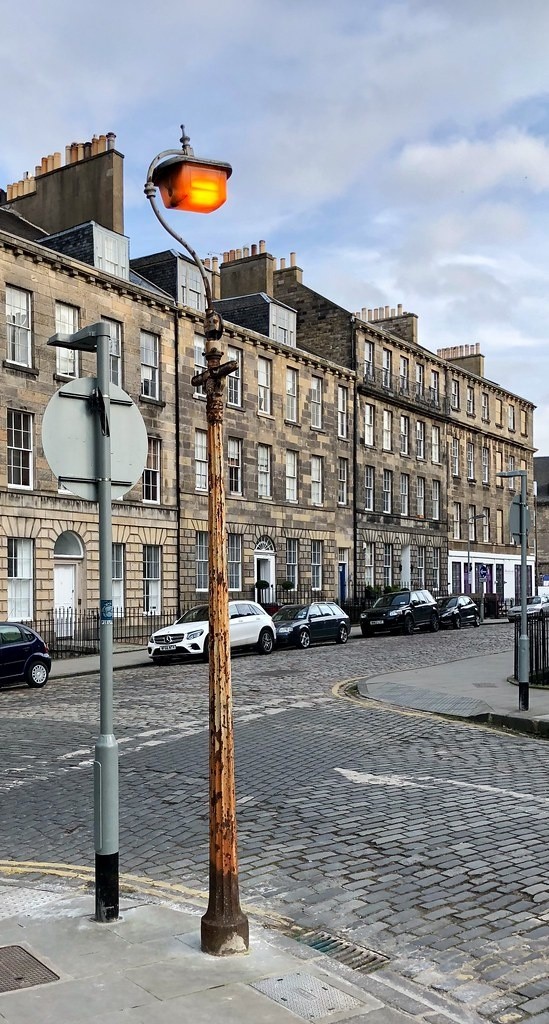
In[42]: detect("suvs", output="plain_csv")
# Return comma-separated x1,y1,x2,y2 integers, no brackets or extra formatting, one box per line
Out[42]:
360,589,440,640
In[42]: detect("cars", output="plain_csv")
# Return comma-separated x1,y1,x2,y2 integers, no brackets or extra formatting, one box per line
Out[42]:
419,595,481,632
1,619,53,689
271,601,352,650
147,598,278,666
506,595,549,622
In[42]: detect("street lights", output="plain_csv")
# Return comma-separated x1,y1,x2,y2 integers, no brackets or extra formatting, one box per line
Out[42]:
144,122,251,959
466,513,486,593
496,468,531,712
